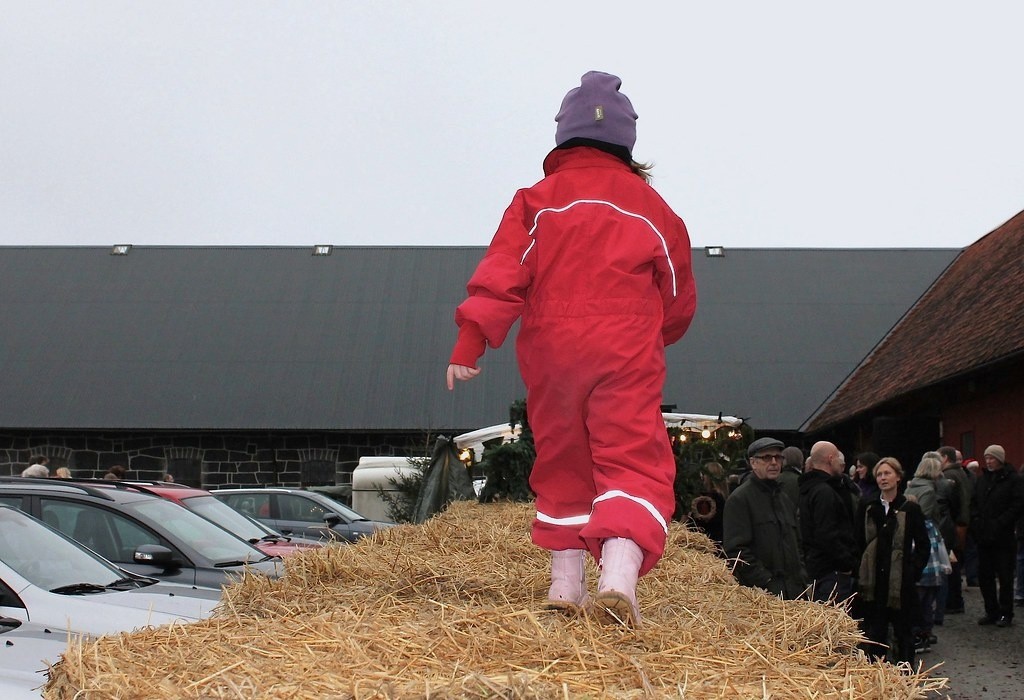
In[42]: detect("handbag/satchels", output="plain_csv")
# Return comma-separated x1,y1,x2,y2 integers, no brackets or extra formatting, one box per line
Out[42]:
938,538,952,575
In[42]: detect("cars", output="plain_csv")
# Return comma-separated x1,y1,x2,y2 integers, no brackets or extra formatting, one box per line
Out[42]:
0,500,227,637
0,614,90,700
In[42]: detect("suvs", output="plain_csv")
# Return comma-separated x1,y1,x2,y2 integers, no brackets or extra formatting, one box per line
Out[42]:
0,473,290,590
73,477,328,565
209,488,401,545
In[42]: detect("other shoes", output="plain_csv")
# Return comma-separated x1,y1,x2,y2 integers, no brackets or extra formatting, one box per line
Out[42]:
925,635,937,644
925,637,932,652
977,615,995,625
967,578,979,587
996,614,1012,627
1014,594,1024,605
914,636,925,653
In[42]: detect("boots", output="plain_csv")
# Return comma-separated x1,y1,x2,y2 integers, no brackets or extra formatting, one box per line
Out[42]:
595,537,644,629
548,549,590,616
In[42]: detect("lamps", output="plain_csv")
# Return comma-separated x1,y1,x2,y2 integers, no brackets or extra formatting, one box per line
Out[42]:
312,245,333,256
706,246,725,258
113,244,132,256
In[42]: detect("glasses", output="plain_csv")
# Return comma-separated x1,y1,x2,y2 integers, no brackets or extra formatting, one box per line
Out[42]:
753,454,784,463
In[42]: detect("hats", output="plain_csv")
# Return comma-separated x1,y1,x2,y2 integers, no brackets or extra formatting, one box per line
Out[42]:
984,445,1005,464
963,458,980,470
555,71,639,156
747,437,784,457
782,447,803,467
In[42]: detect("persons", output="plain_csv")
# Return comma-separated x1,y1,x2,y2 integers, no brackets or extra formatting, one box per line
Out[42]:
674,437,1024,681
21,456,72,479
103,465,127,480
446,70,698,631
163,473,175,483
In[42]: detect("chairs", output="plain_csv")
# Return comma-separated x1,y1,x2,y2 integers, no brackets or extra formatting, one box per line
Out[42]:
41,510,59,529
73,510,104,557
